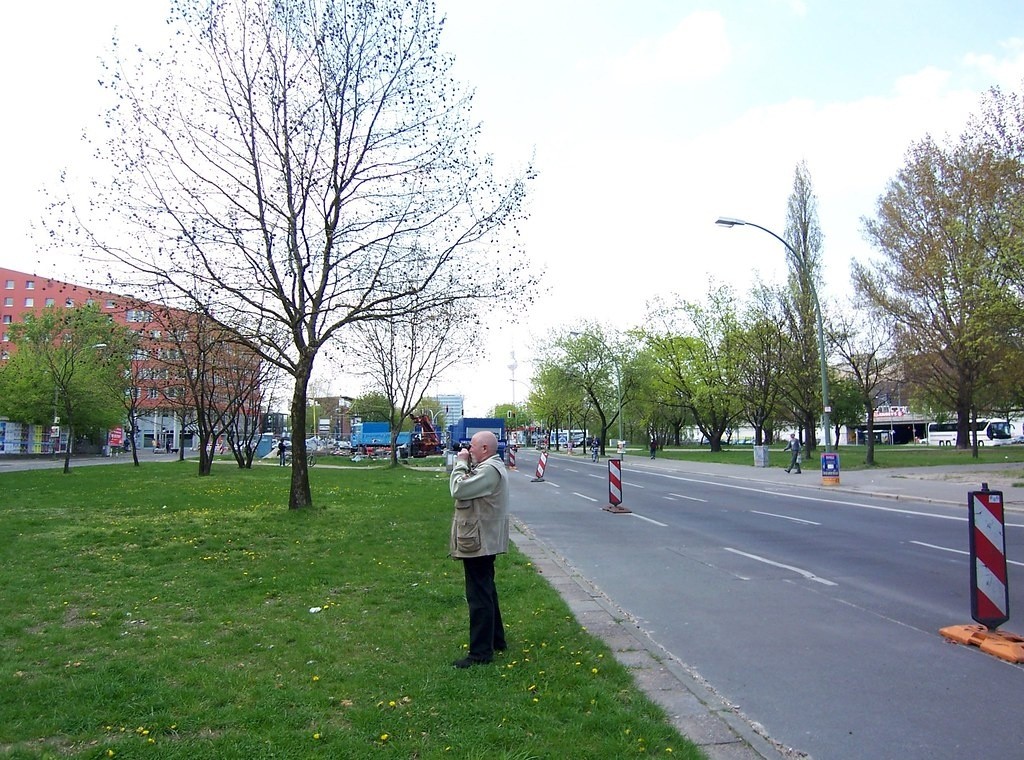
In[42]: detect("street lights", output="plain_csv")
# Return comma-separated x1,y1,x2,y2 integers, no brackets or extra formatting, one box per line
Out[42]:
715,216,832,454
876,395,893,445
570,330,621,461
50,344,108,461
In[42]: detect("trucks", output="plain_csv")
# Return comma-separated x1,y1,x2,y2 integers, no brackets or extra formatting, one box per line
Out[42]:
447,417,507,462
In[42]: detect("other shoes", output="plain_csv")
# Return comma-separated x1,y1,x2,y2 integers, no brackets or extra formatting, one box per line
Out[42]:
454,658,487,668
795,471,801,474
784,469,790,474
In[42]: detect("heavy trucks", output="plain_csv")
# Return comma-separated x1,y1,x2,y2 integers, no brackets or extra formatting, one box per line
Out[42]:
536,428,589,452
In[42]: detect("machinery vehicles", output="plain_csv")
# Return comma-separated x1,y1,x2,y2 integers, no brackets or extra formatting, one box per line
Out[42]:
409,413,446,458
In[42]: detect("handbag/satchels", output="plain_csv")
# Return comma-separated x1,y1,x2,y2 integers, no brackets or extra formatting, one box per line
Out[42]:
796,453,801,463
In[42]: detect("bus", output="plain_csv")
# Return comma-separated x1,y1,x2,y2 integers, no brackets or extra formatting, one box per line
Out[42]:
928,417,1016,448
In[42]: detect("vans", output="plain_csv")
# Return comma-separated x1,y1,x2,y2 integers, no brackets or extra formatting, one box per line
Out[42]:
205,444,219,452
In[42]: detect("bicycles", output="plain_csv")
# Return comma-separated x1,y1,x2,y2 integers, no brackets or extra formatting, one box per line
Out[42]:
590,443,599,463
306,445,317,467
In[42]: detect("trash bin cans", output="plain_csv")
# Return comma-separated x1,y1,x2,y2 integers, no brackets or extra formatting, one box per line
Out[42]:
820,453,840,485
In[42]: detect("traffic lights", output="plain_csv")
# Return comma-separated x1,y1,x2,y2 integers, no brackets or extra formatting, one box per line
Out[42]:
513,411,515,418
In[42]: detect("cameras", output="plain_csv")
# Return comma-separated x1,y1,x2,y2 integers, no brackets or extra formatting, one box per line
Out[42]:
451,441,471,451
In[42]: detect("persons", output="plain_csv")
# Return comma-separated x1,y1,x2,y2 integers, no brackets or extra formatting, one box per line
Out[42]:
449,431,510,669
166,439,171,453
253,440,258,459
220,442,225,455
152,439,161,452
784,434,801,474
544,436,547,449
278,439,287,466
590,437,600,462
650,438,656,459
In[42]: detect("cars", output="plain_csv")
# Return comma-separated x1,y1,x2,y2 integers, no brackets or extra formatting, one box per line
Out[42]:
234,440,256,452
1011,434,1024,444
702,436,754,445
268,433,353,455
